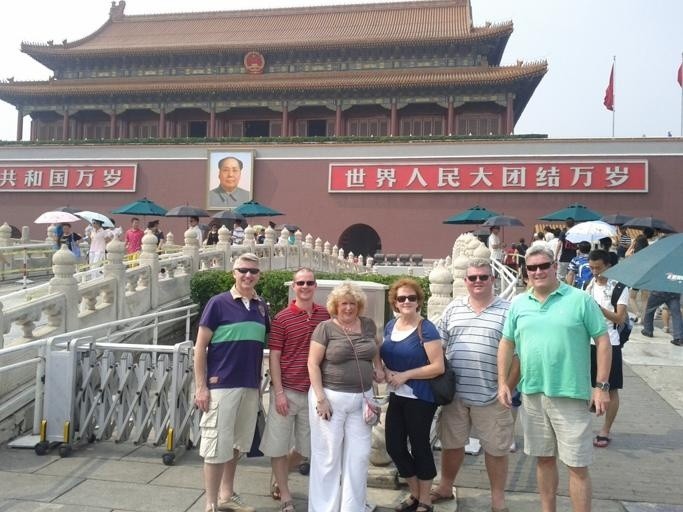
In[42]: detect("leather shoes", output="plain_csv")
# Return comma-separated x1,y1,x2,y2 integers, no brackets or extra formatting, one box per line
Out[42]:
671,340,681,346
641,329,653,337
634,312,641,322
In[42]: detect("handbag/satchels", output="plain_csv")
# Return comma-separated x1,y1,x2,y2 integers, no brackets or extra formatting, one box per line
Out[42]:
417,318,455,405
362,397,381,426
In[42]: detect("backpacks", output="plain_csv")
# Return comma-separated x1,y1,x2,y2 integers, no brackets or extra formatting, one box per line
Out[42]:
574,256,594,290
583,280,631,346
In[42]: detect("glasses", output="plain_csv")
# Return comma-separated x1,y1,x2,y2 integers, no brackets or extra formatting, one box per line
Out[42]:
527,261,555,271
235,268,260,274
466,274,491,281
395,295,418,302
294,280,315,286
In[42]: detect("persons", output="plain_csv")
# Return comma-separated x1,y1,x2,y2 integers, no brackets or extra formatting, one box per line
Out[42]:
305,280,385,511
55,217,299,269
210,155,251,210
492,244,612,512
421,254,523,512
190,252,274,510
578,249,630,448
487,216,682,351
372,279,449,511
258,267,331,511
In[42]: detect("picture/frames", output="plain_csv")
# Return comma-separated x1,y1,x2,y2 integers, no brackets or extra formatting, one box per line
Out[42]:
206,148,254,211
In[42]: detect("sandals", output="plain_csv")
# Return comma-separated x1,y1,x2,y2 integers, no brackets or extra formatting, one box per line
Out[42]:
271,480,281,499
281,500,296,512
394,494,419,512
415,503,434,512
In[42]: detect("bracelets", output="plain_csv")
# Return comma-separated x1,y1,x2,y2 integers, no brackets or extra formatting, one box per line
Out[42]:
316,395,327,403
274,390,284,398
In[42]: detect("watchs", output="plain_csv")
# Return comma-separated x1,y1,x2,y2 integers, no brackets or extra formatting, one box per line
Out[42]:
593,381,611,392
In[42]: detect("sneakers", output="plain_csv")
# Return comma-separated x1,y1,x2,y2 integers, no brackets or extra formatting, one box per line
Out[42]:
217,493,256,512
206,503,218,512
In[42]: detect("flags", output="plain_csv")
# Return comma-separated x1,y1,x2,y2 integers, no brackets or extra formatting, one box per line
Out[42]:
677,63,682,88
602,62,614,112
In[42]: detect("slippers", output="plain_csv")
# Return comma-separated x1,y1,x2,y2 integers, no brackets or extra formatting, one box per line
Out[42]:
593,432,612,447
429,491,455,504
491,506,509,512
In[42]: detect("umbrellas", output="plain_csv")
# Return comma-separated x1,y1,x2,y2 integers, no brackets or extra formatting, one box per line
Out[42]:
597,230,683,299
34,196,286,241
441,207,679,247
